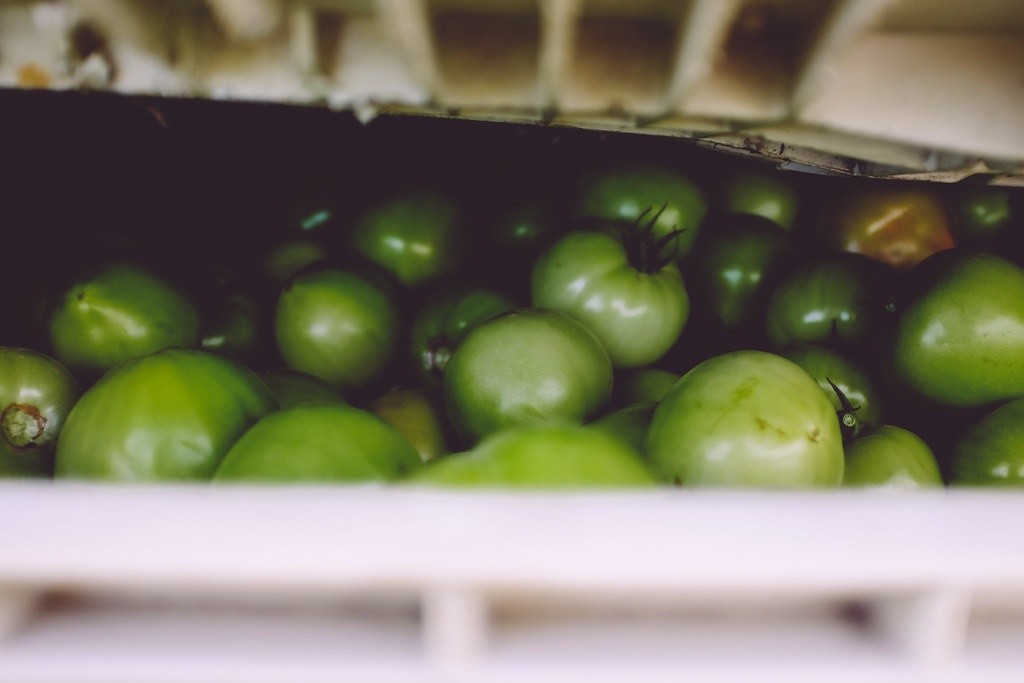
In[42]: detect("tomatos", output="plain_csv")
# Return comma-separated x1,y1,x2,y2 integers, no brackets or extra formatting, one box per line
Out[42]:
0,157,1024,493
